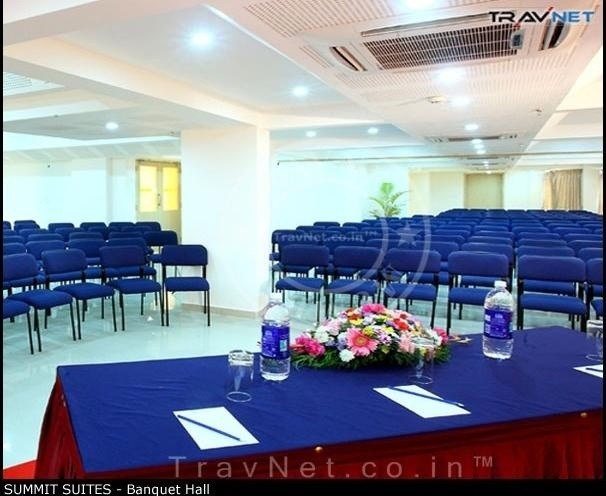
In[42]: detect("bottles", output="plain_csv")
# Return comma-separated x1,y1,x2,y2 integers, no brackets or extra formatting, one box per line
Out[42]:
258,292,293,382
483,279,514,360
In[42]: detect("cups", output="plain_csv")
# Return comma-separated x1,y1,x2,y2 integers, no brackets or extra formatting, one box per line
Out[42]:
586,320,604,364
409,338,435,385
227,350,254,402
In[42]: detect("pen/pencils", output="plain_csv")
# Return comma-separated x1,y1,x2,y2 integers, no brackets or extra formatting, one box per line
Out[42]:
387,384,469,412
586,368,603,372
177,414,240,441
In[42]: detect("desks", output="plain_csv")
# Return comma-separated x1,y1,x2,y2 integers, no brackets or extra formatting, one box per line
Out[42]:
29,322,602,479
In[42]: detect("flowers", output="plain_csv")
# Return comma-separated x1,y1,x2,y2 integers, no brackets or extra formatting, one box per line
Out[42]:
288,301,450,372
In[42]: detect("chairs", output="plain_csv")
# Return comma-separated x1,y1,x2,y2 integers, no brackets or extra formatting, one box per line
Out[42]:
3,217,211,355
269,206,604,332
